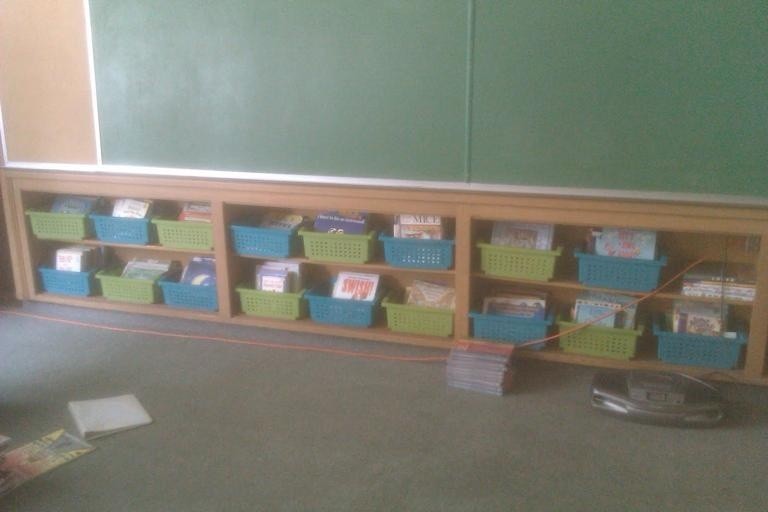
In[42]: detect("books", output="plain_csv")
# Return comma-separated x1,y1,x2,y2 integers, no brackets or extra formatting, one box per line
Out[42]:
55,244,217,287
259,210,444,240
257,260,457,308
0,431,96,496
484,287,731,336
492,220,655,260
50,194,213,222
683,262,756,302
67,392,153,439
446,336,516,394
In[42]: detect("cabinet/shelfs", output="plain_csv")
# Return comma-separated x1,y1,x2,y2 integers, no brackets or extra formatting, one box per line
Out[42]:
2,167,767,386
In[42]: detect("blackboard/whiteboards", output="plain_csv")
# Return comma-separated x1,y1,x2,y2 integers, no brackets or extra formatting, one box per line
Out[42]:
0,0,768,209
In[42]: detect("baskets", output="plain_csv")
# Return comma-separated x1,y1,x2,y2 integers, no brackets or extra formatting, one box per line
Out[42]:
476,237,564,283
228,215,299,257
556,312,645,360
379,228,456,269
298,224,379,264
24,205,215,251
306,277,381,328
37,262,217,310
466,308,554,352
382,287,456,338
234,276,308,321
652,324,748,369
573,242,669,292
